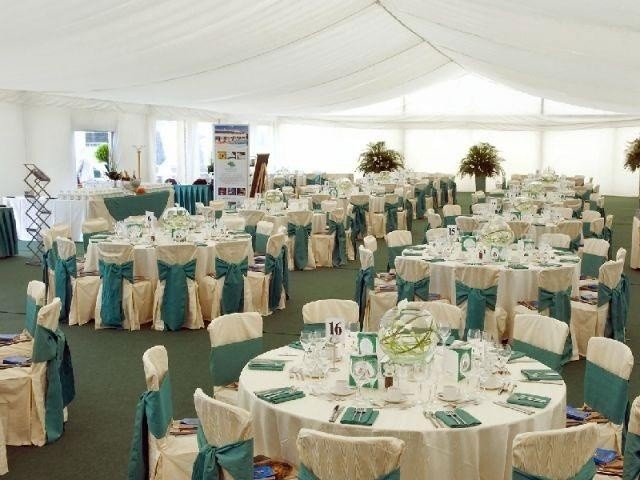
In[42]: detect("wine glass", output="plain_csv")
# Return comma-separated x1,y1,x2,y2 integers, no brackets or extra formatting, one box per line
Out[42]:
299,320,513,407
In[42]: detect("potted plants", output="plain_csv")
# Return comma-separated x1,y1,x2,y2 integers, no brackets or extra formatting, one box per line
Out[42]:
457,141,505,194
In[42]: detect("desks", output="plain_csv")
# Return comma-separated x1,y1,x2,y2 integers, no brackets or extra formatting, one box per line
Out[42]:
1,169,330,256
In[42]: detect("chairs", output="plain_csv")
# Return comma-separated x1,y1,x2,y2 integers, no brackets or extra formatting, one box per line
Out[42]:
303,299,361,341
511,423,599,480
297,428,407,477
207,312,266,402
38,171,458,332
2,280,46,361
351,167,630,362
0,295,76,446
561,336,634,455
595,394,639,479
129,344,200,479
188,387,297,478
